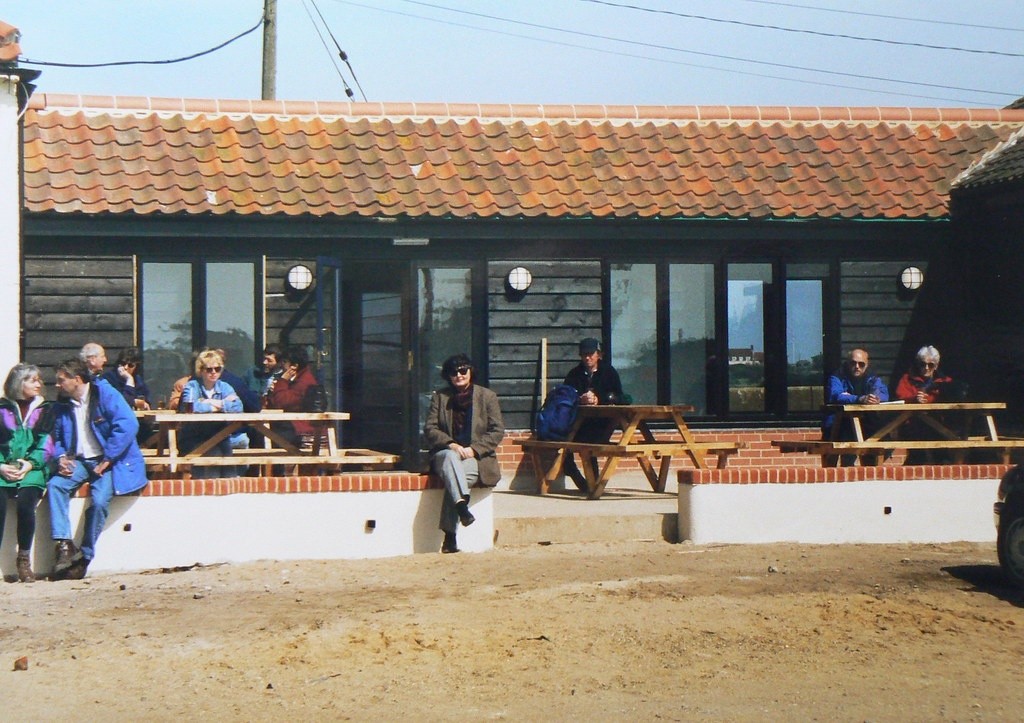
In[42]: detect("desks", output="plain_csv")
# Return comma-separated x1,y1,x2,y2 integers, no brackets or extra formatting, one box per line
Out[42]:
834,403,1009,464
542,405,709,500
148,406,351,480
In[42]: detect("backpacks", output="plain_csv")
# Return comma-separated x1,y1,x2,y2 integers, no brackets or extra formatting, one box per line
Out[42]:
533,383,580,444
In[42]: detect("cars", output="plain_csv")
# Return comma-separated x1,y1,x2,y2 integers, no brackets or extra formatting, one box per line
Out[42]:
992,457,1024,592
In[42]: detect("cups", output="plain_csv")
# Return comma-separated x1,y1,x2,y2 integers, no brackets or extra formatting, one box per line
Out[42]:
9,460,20,480
157,394,166,409
64,455,74,477
184,401,194,413
586,388,595,405
137,394,145,410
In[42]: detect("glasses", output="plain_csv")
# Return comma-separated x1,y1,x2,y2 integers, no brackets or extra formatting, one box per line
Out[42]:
450,367,470,378
120,360,136,368
204,366,223,372
850,360,866,369
920,362,936,369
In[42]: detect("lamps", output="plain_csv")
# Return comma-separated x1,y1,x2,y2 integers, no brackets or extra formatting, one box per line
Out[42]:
504,265,532,297
287,264,314,297
899,265,925,296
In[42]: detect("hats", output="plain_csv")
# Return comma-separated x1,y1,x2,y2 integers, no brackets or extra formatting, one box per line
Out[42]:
579,337,602,356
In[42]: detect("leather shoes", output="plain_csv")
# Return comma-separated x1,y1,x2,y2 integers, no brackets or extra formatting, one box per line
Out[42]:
52,540,84,574
61,557,91,581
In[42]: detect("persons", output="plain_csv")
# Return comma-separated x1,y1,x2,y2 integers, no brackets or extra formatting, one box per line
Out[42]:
45,360,149,579
168,343,327,478
107,346,148,410
563,337,623,476
0,363,56,583
820,349,896,468
424,355,504,552
80,342,106,374
893,345,953,465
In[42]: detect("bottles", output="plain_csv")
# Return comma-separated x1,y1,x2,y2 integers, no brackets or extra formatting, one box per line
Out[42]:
260,391,266,409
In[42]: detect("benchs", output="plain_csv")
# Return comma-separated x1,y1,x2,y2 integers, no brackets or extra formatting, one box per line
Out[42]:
143,448,401,473
766,437,1024,465
516,437,745,501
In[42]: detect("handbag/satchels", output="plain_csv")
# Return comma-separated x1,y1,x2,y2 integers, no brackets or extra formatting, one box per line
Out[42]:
303,384,328,414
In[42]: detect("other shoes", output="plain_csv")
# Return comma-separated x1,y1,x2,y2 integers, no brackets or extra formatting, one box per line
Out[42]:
441,542,460,554
459,511,476,527
14,555,36,584
